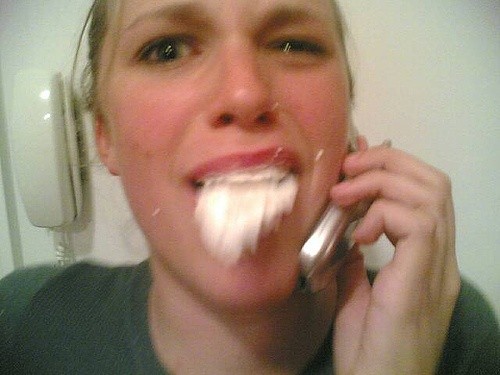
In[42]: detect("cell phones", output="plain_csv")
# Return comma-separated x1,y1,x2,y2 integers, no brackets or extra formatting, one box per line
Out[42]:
294,119,393,297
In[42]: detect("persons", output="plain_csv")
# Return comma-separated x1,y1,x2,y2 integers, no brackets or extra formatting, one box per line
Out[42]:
1,1,499,375
